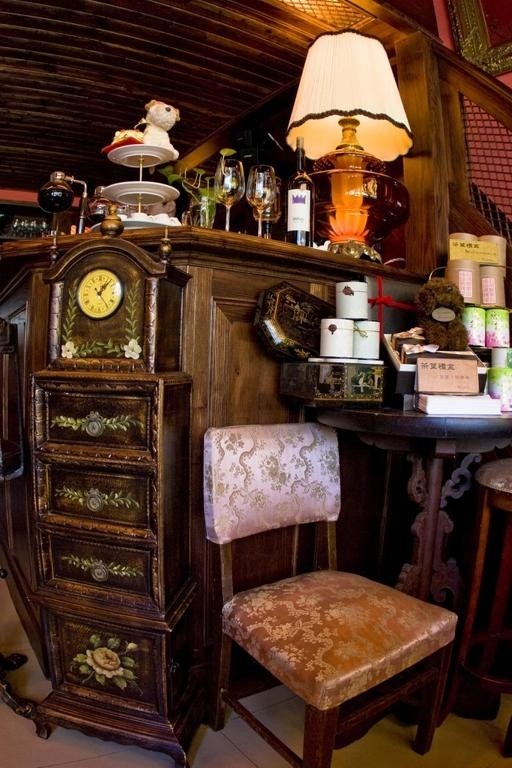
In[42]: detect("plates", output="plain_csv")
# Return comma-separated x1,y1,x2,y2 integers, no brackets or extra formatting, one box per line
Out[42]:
107,143,175,168
90,218,168,233
100,179,181,205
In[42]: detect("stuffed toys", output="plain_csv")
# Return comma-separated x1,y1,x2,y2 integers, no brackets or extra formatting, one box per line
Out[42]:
140,99,181,174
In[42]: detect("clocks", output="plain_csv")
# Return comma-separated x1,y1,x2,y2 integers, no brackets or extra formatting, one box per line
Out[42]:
43,204,193,375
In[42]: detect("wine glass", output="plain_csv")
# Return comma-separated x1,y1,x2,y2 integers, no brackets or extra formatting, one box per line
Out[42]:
251,175,282,241
214,157,246,231
245,163,277,239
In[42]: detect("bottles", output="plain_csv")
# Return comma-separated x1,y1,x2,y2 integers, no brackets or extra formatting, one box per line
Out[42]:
9,217,49,239
282,134,317,248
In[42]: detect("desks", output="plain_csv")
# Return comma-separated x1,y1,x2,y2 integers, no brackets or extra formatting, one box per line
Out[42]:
300,410,512,729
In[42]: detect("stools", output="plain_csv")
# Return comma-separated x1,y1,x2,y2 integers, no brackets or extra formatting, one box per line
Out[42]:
438,457,512,754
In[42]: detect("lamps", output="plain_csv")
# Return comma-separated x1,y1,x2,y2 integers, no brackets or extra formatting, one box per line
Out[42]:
285,30,417,261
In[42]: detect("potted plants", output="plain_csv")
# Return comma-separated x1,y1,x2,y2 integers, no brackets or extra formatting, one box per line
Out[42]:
160,148,239,229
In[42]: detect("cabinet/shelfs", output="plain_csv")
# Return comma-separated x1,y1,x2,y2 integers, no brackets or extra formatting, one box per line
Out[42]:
23,371,198,768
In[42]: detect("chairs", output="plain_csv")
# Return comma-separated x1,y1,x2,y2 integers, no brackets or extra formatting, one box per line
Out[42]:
200,424,460,768
0,318,53,718
